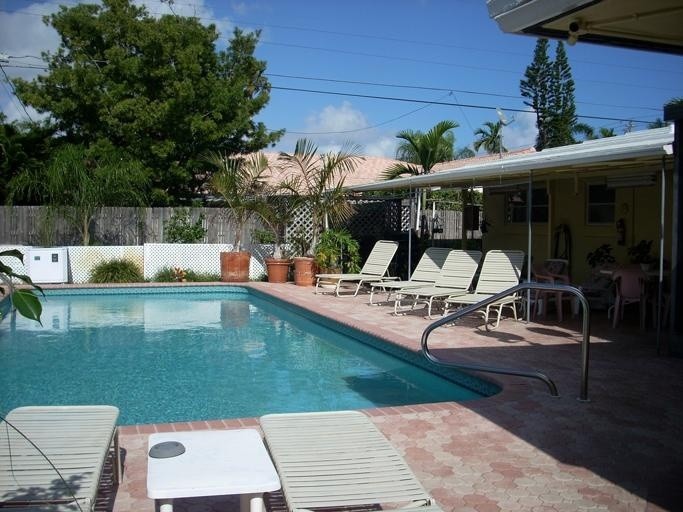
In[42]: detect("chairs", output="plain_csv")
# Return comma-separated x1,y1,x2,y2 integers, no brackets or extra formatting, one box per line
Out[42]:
313,239,574,333
256,408,443,512
2,405,123,512
611,264,648,328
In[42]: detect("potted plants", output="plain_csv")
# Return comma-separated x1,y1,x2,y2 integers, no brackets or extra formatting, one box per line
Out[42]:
627,240,653,271
198,141,361,285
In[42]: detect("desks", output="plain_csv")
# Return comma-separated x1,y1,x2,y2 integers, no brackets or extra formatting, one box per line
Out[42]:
147,429,280,512
599,267,670,331
